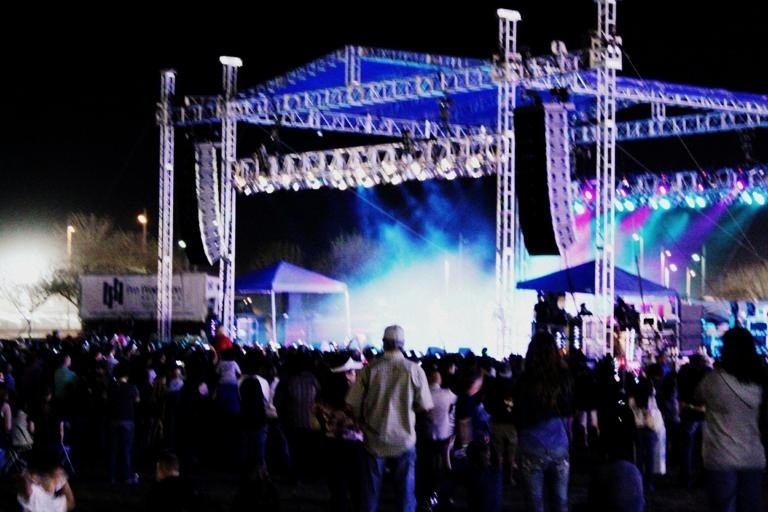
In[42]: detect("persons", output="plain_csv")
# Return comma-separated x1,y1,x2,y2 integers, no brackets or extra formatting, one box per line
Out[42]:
1,292,768,512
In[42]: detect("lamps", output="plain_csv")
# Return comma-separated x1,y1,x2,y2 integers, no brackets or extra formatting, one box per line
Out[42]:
231,125,498,195
435,95,453,121
567,163,768,216
269,114,283,143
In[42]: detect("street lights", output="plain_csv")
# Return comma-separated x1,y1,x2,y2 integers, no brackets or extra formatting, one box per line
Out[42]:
632,232,644,280
66,224,76,272
690,244,706,297
664,263,678,289
686,267,696,298
138,214,149,262
660,245,672,289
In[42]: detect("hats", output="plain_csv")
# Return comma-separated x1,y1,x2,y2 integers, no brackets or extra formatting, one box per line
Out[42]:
385,325,404,341
